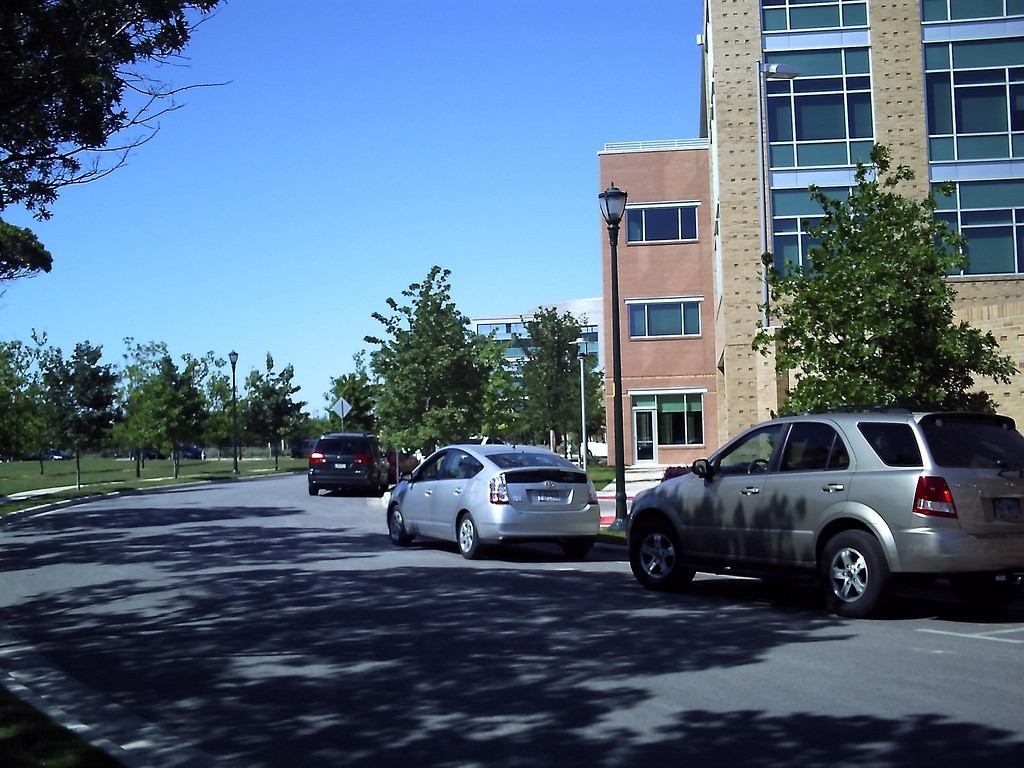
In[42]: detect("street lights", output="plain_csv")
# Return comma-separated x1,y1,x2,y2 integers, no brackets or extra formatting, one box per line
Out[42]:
228,349,242,475
597,181,629,531
568,338,589,471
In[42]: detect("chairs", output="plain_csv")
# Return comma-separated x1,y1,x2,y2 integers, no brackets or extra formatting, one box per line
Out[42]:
802,446,831,469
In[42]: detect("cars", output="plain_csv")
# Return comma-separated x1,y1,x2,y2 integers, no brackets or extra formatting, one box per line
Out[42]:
377,438,421,484
291,435,325,460
387,444,600,560
0,446,70,462
438,434,510,472
129,442,207,460
628,409,1024,618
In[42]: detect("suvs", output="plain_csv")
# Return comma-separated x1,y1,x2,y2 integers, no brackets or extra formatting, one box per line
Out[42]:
308,431,390,496
